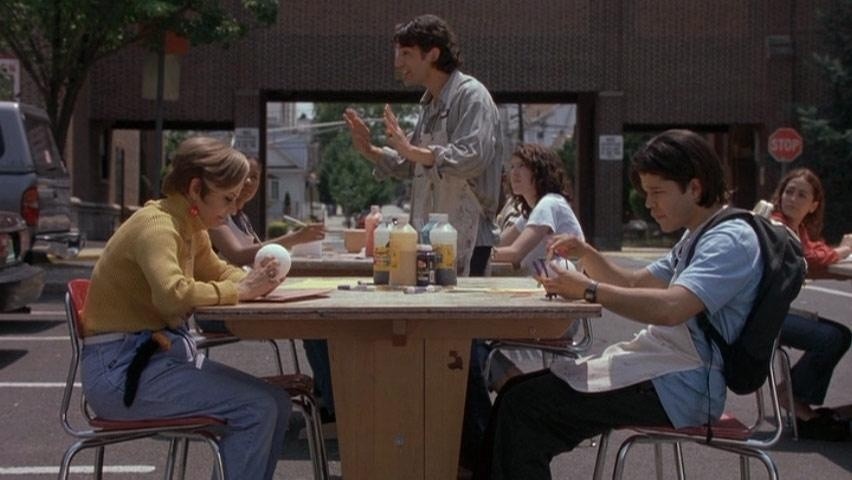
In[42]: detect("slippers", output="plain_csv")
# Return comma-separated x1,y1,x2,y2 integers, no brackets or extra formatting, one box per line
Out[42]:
791,409,851,442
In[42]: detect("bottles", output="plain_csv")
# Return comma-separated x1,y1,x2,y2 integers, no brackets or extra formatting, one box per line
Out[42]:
364,203,458,288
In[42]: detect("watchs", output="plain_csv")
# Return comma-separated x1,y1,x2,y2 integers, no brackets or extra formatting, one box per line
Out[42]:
583,279,598,304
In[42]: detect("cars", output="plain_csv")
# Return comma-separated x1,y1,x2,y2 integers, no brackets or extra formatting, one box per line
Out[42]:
351,206,372,231
0,209,47,314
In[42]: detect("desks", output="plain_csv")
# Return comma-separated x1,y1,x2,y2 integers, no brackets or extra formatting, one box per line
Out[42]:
195,276,603,480
284,240,512,277
828,263,852,276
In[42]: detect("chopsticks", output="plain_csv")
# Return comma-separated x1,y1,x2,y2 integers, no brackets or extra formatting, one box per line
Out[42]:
531,256,557,301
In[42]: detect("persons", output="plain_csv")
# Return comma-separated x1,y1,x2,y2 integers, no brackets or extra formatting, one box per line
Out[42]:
193,151,334,422
79,137,293,479
479,129,766,480
474,141,586,396
766,168,852,440
342,15,503,472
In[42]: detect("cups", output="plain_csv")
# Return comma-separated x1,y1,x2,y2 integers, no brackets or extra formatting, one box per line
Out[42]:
343,229,365,254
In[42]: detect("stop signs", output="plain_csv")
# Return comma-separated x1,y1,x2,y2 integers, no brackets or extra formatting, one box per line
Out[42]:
768,128,805,165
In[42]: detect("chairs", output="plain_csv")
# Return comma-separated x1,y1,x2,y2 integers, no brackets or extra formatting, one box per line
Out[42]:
58,279,330,480
482,220,807,480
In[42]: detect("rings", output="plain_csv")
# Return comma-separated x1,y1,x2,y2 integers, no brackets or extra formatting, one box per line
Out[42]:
269,270,274,277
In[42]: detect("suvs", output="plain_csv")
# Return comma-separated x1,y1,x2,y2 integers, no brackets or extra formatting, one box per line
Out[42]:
0,96,89,265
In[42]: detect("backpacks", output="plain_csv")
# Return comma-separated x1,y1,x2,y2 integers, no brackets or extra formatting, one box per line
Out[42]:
676,207,809,395
